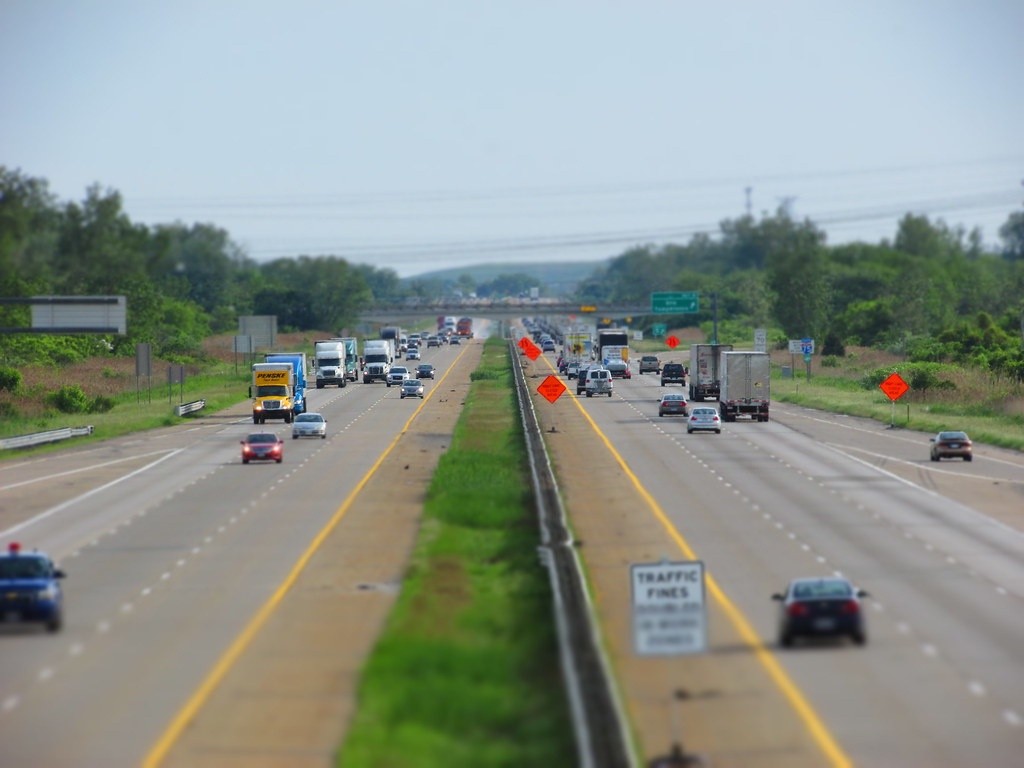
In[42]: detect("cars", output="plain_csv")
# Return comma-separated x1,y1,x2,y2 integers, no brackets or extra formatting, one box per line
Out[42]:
929,429,972,461
522,316,563,351
0,543,66,633
415,364,435,379
292,414,327,439
658,364,686,387
683,408,723,434
657,394,690,418
771,577,868,647
399,379,425,399
637,357,661,375
240,432,283,464
400,328,459,361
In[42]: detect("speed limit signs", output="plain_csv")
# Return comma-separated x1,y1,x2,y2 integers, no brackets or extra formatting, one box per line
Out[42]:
756,328,766,345
802,344,813,354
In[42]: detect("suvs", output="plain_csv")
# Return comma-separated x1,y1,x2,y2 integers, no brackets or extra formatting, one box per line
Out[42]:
385,366,410,387
557,355,631,398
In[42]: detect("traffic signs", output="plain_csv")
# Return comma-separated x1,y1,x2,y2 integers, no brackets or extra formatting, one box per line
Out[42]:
651,290,701,314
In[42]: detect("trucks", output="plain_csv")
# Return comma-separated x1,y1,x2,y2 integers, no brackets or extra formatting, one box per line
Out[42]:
715,351,769,422
360,327,400,383
561,328,628,362
437,316,473,339
685,344,734,401
312,338,358,389
249,352,307,424
